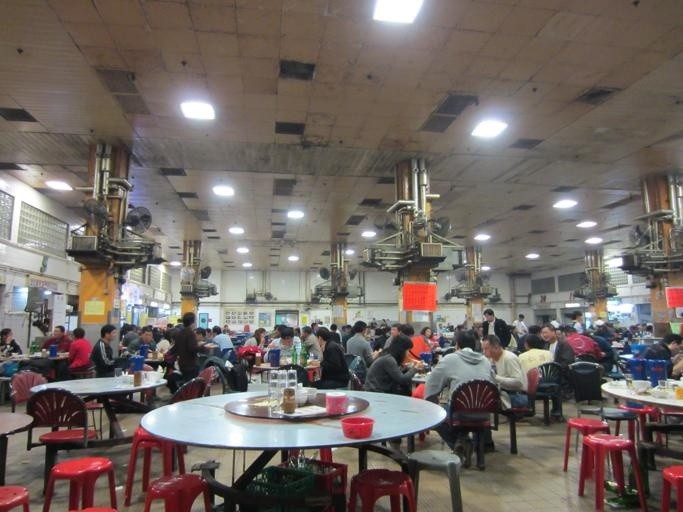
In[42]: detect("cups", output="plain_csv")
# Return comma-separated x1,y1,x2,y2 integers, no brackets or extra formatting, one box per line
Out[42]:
0,353,6,360
270,349,279,366
114,368,130,388
267,368,298,400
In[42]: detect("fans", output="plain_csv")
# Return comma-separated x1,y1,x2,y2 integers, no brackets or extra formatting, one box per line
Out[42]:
347,268,357,280
121,206,152,237
432,216,451,238
630,223,651,247
83,198,109,236
320,267,332,281
198,266,212,280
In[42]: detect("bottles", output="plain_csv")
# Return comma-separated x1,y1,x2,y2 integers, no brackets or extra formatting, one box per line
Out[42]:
282,387,296,414
129,342,164,360
623,357,683,400
29,341,57,357
291,342,313,368
133,371,142,386
254,352,261,367
286,448,329,474
410,352,439,377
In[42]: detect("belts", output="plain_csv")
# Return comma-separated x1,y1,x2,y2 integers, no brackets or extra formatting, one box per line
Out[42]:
501,388,528,395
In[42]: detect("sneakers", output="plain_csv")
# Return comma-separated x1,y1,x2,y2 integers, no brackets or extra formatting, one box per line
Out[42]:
463,440,474,468
548,415,565,423
452,446,464,464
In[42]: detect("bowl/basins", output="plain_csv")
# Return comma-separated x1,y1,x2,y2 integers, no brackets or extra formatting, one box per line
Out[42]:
341,417,376,440
58,351,70,359
324,390,350,415
141,371,164,382
11,353,26,359
296,387,318,405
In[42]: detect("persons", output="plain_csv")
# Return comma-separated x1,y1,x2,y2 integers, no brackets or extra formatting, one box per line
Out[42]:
0,313,323,402
309,318,492,466
482,310,683,422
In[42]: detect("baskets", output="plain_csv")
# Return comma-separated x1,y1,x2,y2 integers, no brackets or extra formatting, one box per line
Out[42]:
239,457,347,511
